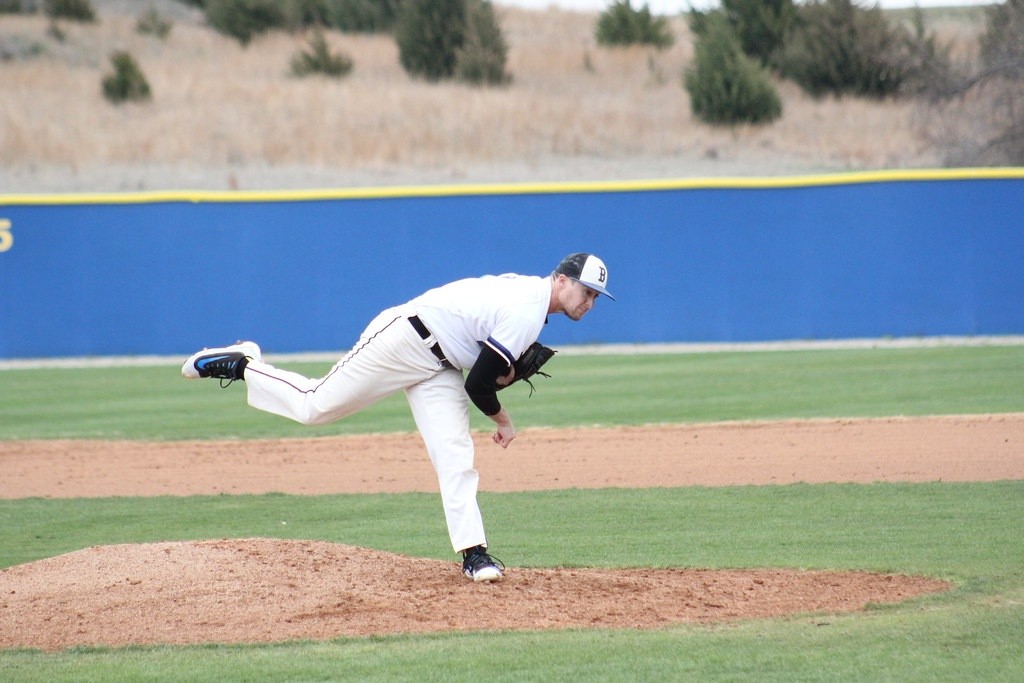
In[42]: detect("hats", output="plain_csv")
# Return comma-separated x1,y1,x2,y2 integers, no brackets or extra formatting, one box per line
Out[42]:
554,253,616,301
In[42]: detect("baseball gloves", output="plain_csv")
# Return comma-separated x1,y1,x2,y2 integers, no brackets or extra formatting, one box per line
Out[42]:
495,341,559,399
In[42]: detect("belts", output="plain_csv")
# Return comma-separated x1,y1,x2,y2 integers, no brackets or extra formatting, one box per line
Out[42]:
407,315,453,368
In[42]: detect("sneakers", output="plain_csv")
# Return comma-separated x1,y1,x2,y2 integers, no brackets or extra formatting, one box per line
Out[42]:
181,340,261,389
461,545,505,582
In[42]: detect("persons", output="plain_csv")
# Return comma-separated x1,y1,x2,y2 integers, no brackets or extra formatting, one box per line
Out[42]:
182,253,615,581
